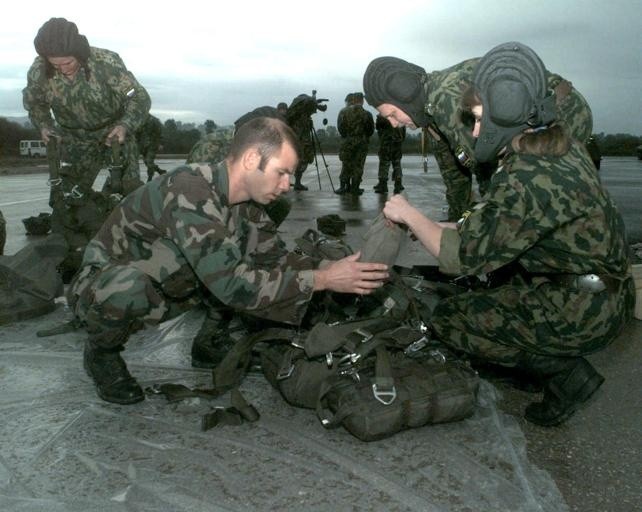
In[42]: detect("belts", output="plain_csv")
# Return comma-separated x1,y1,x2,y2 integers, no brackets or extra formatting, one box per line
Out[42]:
548,273,608,296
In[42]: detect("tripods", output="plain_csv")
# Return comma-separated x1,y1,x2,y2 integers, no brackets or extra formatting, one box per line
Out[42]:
306,121,335,190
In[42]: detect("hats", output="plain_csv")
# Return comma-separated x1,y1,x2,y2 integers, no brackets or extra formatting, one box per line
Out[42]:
472,41,556,165
363,56,435,128
34,18,91,81
234,106,289,136
344,92,365,101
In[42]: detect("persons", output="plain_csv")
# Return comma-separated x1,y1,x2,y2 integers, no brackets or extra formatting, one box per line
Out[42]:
134,114,166,183
66,115,390,406
381,40,636,428
362,55,602,293
22,17,152,286
184,106,290,230
277,94,406,194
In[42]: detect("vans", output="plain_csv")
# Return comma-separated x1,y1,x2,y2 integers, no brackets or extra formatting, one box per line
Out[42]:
20,137,48,159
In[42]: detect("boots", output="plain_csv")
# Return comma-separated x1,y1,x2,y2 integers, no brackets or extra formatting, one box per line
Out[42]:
335,176,364,194
83,337,145,405
294,175,308,190
190,307,266,371
517,349,604,429
373,178,403,194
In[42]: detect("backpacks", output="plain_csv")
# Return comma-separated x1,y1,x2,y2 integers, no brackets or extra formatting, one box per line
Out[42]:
47,135,125,268
141,316,481,443
